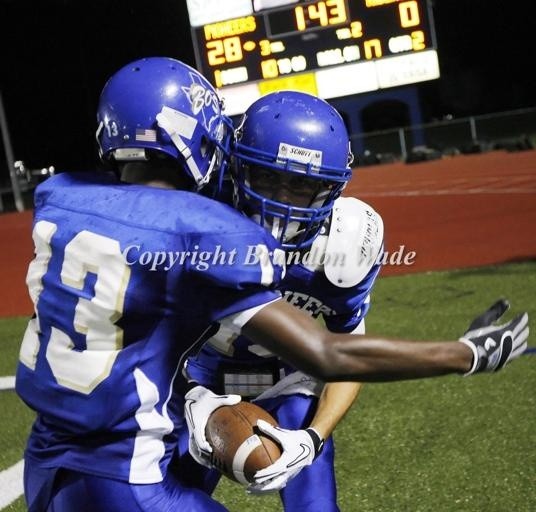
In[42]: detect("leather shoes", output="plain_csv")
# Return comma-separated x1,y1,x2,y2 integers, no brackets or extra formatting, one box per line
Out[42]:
181,382,243,473
454,297,531,379
243,418,326,497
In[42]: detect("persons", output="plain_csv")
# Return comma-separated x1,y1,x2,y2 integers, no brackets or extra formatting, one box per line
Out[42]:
14,55,531,511
166,90,385,512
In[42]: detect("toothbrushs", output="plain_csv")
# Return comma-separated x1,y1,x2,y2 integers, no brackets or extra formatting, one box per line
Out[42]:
201,402,283,485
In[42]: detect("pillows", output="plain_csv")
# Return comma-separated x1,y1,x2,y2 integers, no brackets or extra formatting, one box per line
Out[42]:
228,88,354,254
91,54,235,200
